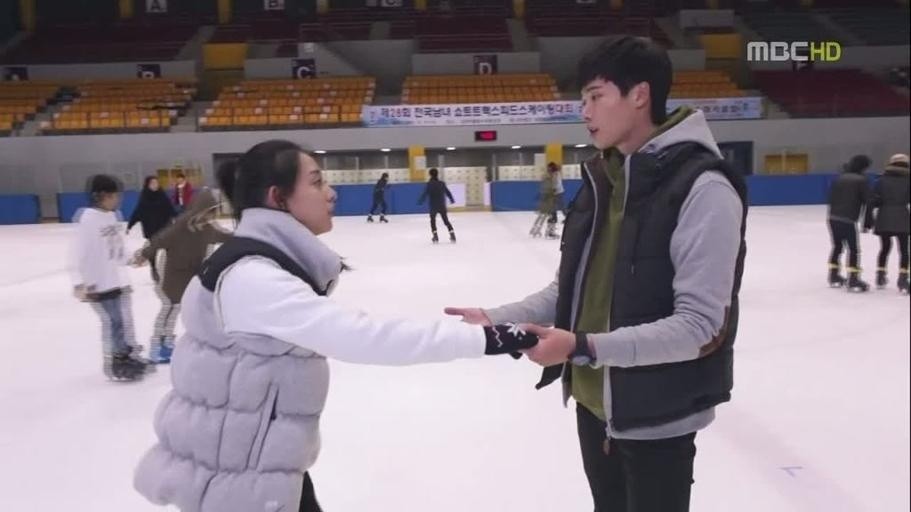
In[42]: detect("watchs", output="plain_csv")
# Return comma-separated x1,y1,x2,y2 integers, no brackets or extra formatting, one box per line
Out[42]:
565,331,593,368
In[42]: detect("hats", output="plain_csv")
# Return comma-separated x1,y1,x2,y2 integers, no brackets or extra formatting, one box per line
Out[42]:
891,154,910,164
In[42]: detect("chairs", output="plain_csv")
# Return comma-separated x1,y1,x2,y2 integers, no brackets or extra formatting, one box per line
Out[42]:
1,74,204,135
2,0,911,67
191,72,565,134
655,64,911,120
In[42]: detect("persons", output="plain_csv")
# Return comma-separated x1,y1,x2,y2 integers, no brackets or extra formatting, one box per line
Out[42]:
530,160,563,241
861,153,910,292
73,173,232,382
444,34,749,512
414,165,456,240
365,173,389,223
131,139,536,512
824,154,871,288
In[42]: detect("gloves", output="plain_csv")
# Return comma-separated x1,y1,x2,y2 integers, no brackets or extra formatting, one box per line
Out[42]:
483,318,539,356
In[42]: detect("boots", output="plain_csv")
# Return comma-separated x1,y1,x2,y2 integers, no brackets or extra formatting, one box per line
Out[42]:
830,269,910,291
